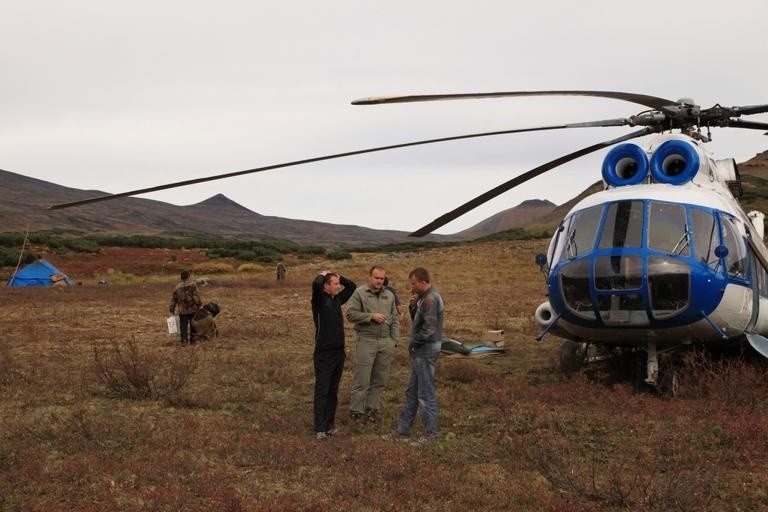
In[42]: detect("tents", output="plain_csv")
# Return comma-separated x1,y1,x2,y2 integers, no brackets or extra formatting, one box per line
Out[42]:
7,258,75,289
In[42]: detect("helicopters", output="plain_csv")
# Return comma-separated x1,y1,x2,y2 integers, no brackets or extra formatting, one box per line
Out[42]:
44,89,766,399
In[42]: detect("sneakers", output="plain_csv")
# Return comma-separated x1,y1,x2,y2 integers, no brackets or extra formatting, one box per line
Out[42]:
348,408,378,427
380,431,430,448
315,428,339,442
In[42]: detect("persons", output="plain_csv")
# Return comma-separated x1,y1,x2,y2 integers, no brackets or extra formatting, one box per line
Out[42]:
274,261,287,280
378,267,445,448
169,271,202,349
382,275,403,321
344,263,402,427
310,268,357,442
